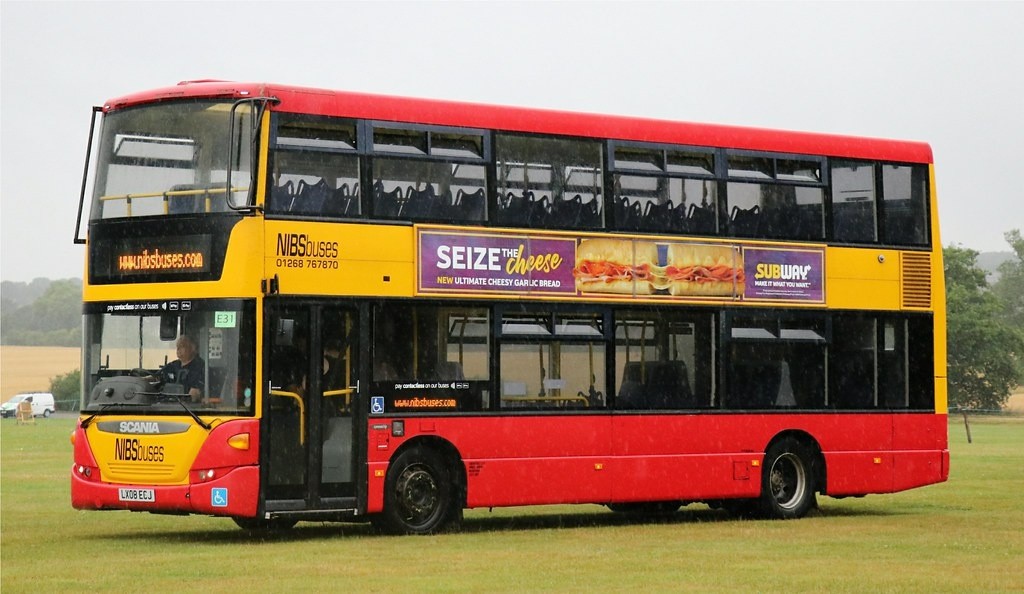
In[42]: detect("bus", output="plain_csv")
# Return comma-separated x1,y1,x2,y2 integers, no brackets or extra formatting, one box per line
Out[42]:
67,77,953,535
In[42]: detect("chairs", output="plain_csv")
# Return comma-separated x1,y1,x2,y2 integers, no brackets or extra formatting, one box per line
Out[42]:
504,352,920,406
163,181,914,246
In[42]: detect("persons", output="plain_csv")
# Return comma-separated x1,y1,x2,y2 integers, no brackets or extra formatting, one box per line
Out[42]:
291,329,399,391
146,334,212,396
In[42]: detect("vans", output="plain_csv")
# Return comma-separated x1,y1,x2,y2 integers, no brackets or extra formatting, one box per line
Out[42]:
0,393,56,418
